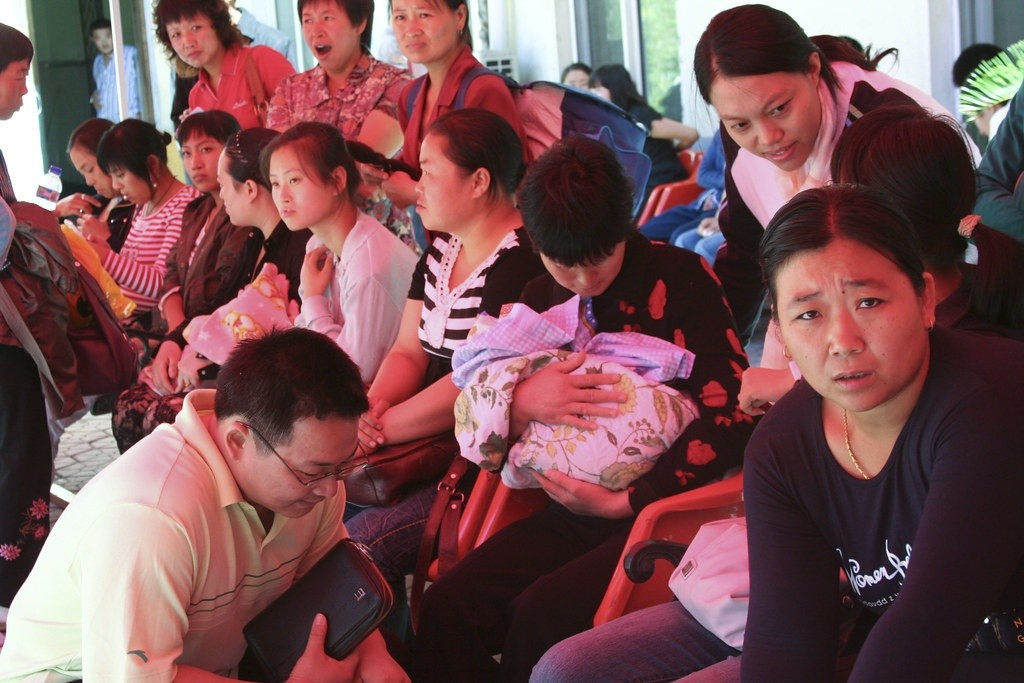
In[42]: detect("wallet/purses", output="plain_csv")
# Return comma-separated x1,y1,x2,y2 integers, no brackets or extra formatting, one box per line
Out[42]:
243,538,394,683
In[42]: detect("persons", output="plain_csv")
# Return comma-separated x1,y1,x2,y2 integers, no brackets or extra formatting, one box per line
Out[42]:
733,187,1023,683
89,17,142,124
0,329,416,683
1,0,1023,683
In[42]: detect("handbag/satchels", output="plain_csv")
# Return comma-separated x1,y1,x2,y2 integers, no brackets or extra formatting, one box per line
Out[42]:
336,433,460,506
668,517,751,652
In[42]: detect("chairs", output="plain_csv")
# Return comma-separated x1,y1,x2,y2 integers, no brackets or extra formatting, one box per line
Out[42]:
641,144,707,226
429,462,746,626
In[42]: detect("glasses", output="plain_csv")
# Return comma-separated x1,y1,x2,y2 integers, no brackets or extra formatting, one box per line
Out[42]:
236,420,370,490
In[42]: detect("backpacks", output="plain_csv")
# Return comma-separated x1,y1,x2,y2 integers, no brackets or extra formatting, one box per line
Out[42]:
404,66,652,223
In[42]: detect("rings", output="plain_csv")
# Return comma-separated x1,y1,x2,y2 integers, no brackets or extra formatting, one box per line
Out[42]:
81,193,86,200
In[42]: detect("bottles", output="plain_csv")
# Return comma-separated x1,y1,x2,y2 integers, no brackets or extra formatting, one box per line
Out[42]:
32,165,63,211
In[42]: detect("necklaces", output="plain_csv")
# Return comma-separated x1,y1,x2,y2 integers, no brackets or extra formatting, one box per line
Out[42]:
841,409,872,480
